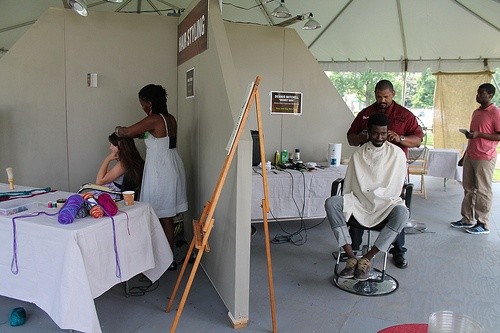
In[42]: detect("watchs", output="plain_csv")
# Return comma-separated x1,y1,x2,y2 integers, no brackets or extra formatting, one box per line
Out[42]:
400,136,405,140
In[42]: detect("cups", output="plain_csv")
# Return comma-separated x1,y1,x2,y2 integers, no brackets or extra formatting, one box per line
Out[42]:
427,310,486,333
121,190,135,205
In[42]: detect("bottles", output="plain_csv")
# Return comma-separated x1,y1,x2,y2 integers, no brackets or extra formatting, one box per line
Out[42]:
275,149,300,166
330,150,337,167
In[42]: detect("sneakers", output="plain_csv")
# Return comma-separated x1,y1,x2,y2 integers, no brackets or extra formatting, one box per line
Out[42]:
340,257,358,278
465,224,490,234
451,220,474,228
355,257,370,281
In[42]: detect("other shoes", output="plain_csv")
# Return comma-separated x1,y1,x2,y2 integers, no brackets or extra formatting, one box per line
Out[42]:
168,262,177,270
332,252,356,262
393,254,407,269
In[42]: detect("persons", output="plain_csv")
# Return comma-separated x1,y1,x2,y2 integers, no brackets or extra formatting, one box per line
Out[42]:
332,80,423,268
450,83,500,233
324,113,410,281
114,84,189,271
96,131,145,201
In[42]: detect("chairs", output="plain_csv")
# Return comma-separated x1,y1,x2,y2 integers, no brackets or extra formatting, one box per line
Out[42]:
329,179,415,296
408,148,429,199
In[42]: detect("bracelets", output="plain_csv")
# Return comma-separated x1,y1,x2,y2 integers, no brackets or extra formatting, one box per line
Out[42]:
115,128,120,137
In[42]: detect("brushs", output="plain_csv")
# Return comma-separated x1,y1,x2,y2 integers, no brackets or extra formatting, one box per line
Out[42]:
6,168,15,189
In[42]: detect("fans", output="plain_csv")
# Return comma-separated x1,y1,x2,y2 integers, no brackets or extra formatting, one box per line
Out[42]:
405,116,428,165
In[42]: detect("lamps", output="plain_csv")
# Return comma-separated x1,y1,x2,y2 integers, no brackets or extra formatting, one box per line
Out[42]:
67,0,90,17
272,0,293,18
279,12,321,30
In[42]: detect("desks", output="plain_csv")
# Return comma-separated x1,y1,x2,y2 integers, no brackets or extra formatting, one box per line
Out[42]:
427,150,463,192
0,183,174,333
251,164,350,223
